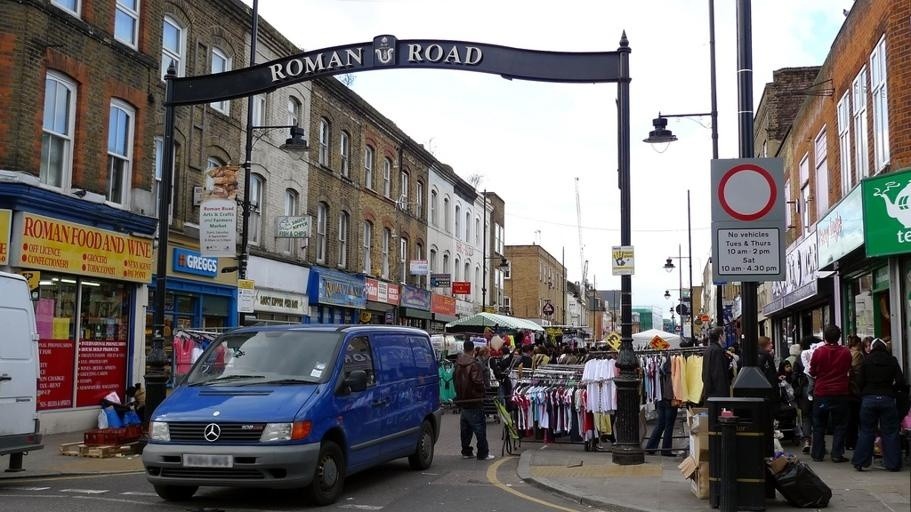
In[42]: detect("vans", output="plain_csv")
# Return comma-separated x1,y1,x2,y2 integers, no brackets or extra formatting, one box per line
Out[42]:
141,322,443,508
1,273,44,456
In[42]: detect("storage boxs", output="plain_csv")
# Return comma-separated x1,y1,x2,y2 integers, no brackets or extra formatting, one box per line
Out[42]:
677,406,710,499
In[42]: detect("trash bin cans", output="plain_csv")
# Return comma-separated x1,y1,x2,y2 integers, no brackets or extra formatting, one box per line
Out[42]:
704,397,776,512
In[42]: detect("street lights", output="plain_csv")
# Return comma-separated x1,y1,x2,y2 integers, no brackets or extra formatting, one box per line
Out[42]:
642,0,724,338
663,189,694,341
220,0,309,324
562,246,578,325
663,244,683,339
482,189,508,312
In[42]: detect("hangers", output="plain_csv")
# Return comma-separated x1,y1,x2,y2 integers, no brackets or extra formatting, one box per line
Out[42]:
517,376,587,390
584,350,701,363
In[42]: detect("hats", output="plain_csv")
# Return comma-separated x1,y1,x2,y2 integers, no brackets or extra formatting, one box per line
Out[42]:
871,338,886,350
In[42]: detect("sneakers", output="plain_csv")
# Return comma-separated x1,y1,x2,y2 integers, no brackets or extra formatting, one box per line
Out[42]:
480,455,495,460
808,395,814,401
661,453,677,457
612,442,617,446
854,464,862,469
802,440,810,453
646,448,655,455
464,453,477,458
813,457,823,461
832,457,849,462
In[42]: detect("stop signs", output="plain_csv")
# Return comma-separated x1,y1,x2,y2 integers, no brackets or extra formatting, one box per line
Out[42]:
716,163,777,222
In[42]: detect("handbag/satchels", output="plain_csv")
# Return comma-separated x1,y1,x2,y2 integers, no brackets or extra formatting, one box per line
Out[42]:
848,381,860,400
775,400,797,429
490,367,499,387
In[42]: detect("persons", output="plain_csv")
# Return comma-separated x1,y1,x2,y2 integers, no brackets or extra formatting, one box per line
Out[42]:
644,357,678,456
452,340,616,460
696,327,731,408
756,322,911,472
